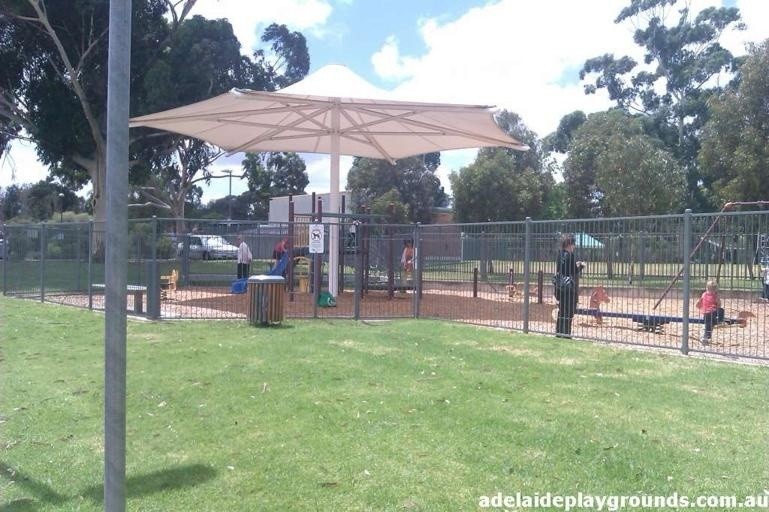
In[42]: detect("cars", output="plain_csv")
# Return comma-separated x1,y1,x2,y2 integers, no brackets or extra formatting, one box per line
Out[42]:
0,230,8,260
174,234,239,260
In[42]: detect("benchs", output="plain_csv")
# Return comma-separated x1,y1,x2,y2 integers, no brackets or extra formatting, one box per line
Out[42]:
88,283,146,313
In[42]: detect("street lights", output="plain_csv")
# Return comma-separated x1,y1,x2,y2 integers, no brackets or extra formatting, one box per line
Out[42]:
221,169,232,227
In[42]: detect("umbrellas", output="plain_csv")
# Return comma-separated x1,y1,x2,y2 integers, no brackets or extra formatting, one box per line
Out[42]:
573,232,604,250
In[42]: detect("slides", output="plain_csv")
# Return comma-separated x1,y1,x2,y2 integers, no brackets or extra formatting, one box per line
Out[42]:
232,246,308,294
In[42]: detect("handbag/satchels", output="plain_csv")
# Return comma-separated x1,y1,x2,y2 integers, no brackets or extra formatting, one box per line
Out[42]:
552,271,575,291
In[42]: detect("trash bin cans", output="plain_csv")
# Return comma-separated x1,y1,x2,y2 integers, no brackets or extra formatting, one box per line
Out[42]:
248,275,285,327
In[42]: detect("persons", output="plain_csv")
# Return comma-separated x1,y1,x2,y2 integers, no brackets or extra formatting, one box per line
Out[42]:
553,232,586,338
695,279,721,346
234,234,253,280
760,257,769,317
399,236,416,292
271,234,289,270
346,216,363,247
128,62,531,301
589,282,611,325
229,191,370,302
